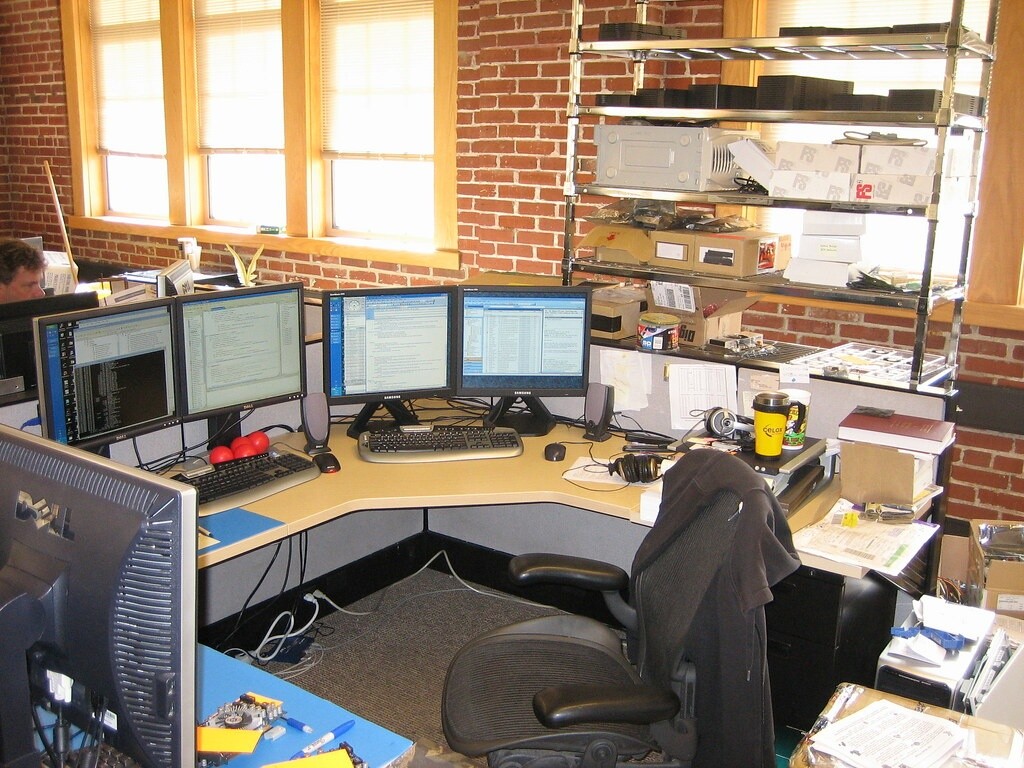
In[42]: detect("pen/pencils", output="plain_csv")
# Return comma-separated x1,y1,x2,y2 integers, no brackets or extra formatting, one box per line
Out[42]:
289,719,355,760
281,714,313,735
199,525,210,536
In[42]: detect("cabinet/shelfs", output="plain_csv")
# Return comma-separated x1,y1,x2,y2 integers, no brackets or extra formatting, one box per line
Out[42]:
558,0,1002,382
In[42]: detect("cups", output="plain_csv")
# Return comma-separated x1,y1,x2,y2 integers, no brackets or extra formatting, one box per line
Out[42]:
177,238,202,270
751,391,806,461
779,388,811,451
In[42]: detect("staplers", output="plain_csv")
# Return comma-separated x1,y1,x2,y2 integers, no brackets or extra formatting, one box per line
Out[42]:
623,432,677,453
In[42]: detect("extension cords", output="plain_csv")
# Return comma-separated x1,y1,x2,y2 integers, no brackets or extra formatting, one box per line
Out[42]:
235,649,255,665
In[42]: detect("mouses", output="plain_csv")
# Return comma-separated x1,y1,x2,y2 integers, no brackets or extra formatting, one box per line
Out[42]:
312,452,341,473
544,442,566,461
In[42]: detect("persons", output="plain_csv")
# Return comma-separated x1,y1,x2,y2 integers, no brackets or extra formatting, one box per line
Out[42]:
0,236,48,304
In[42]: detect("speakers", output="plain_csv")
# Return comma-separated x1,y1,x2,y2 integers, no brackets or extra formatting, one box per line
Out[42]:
299,393,332,455
583,382,615,442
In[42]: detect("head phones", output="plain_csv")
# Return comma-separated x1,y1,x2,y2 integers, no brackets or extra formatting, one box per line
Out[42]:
608,453,665,484
703,407,755,438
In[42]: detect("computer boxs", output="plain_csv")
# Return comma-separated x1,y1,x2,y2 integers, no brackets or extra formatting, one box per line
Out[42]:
875,596,995,711
593,125,759,195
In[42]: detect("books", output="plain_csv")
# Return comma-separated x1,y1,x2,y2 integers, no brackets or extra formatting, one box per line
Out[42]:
891,484,944,512
837,408,956,456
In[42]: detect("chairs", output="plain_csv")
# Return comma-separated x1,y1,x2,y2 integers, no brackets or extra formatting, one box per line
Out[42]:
441,447,801,768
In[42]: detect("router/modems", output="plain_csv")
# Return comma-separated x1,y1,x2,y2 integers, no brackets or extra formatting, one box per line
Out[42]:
268,634,313,664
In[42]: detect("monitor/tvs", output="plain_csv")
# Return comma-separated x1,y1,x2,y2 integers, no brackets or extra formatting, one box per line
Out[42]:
0,259,594,462
0,424,197,768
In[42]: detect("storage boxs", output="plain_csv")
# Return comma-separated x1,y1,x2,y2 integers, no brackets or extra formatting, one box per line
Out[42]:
775,140,862,173
591,282,647,339
644,280,768,346
850,173,934,206
966,519,1024,620
838,442,932,524
768,169,855,202
574,224,792,277
789,681,1024,768
783,211,865,288
860,145,972,178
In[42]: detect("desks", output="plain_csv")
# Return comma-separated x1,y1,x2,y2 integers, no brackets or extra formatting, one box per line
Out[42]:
33,644,418,768
145,397,932,581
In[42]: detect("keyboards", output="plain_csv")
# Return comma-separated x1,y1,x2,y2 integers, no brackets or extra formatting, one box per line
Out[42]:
170,448,321,518
359,425,523,463
